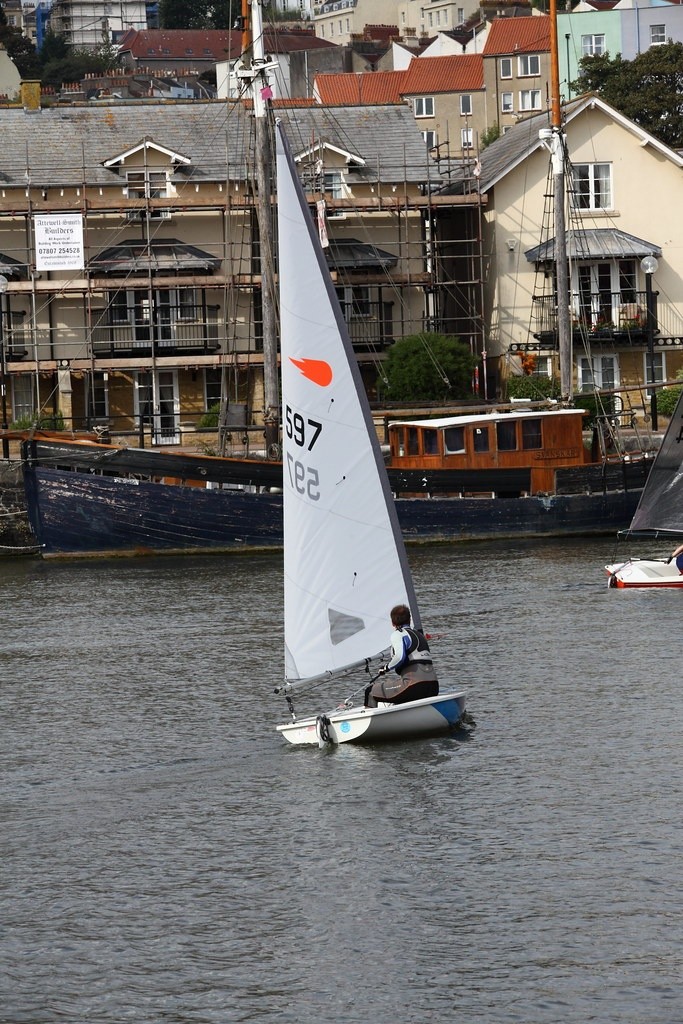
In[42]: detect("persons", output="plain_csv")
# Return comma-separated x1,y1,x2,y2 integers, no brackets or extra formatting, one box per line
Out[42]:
363,604,440,708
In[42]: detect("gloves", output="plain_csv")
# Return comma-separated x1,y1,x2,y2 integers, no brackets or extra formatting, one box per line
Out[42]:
379,663,391,676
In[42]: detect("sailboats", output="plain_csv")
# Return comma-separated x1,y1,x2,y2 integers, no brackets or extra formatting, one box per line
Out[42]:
604,391,682,589
265,117,466,746
0,0,658,559
